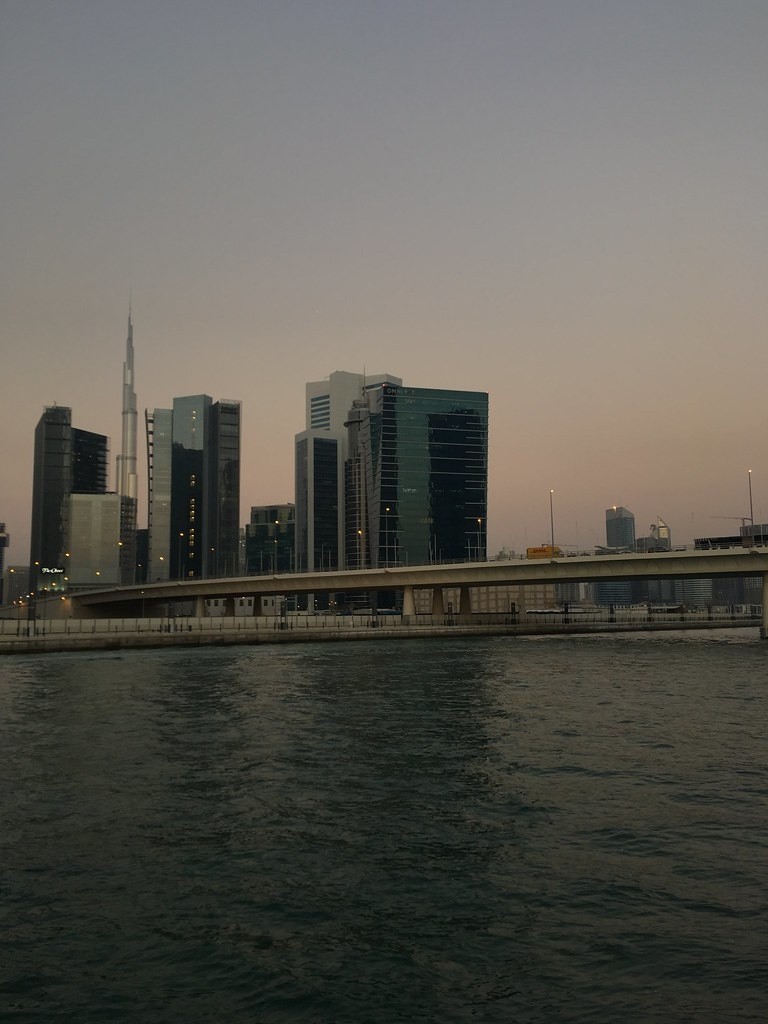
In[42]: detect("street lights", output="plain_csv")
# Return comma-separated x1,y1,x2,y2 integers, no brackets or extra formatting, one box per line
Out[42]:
13,598,22,636
386,508,389,568
550,490,554,557
61,596,70,635
288,503,297,573
179,531,183,579
748,469,754,547
30,591,37,636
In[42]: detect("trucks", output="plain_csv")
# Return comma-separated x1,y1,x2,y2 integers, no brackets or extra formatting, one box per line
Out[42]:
377,605,403,615
527,545,565,558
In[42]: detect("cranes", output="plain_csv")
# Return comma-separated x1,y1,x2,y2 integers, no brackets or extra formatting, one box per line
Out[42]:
709,516,752,526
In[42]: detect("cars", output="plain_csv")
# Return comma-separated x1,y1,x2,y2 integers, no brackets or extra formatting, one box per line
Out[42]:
36,590,49,597
568,553,578,557
648,546,669,553
617,551,632,554
579,553,591,556
336,610,352,615
177,613,190,617
56,592,68,596
